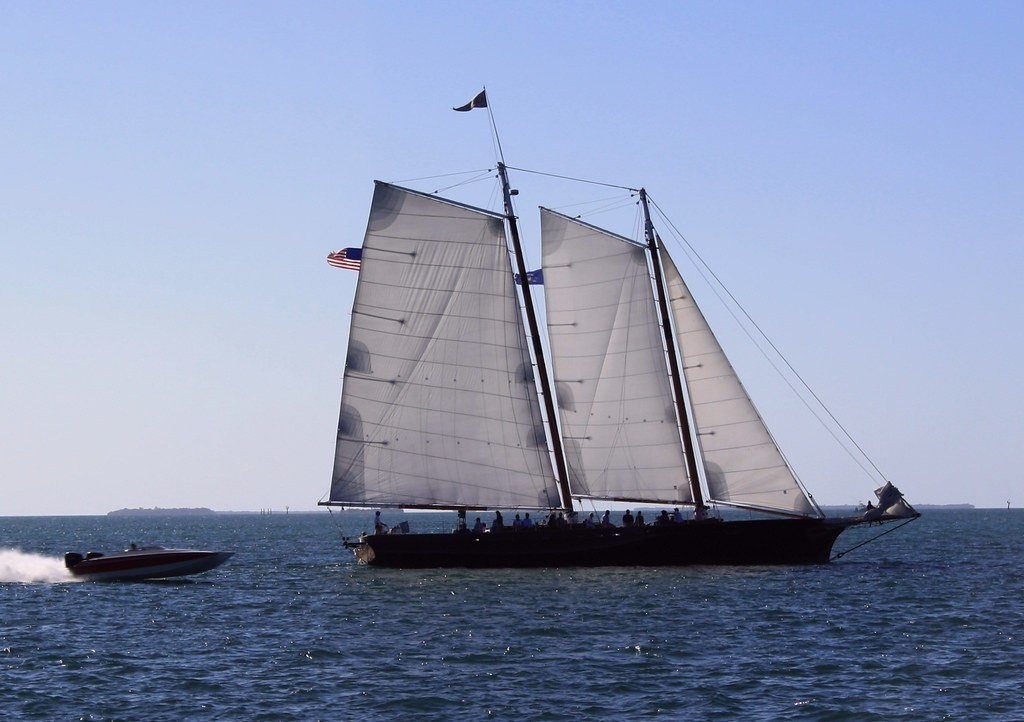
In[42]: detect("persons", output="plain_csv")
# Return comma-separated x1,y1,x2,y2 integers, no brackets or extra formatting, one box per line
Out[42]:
866,501,882,527
473,506,709,532
374,511,387,536
130,544,138,551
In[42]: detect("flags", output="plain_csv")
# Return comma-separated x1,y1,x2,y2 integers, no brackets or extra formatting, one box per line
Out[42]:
453,90,488,111
326,248,362,271
515,269,544,285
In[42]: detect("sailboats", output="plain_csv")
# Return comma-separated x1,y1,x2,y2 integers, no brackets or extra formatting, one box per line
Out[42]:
317,163,924,568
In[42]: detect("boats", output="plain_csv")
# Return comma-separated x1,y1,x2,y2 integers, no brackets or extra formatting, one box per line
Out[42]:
65,541,238,581
